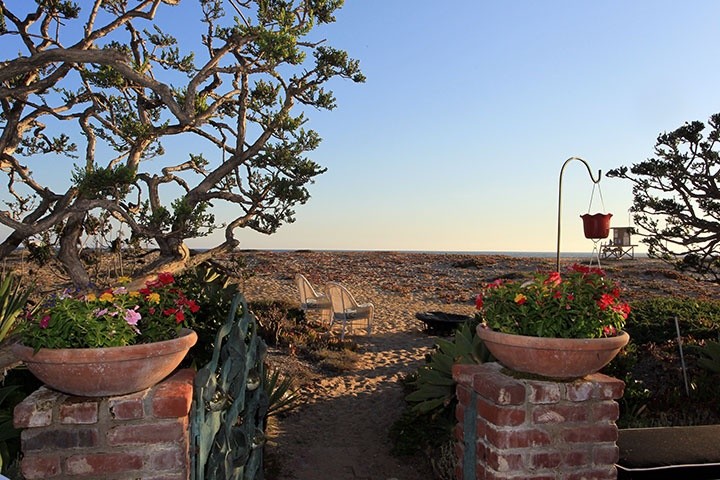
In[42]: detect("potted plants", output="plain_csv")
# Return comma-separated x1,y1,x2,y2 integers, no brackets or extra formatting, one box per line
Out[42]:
618,403,720,466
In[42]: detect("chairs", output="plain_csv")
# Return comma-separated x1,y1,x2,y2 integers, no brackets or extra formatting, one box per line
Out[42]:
325,282,374,341
295,273,333,326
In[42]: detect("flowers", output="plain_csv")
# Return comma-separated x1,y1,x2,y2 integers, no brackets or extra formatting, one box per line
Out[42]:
474,271,630,336
14,272,202,342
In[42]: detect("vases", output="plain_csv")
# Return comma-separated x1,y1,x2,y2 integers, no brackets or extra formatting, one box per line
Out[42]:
580,213,614,239
476,324,630,379
12,328,198,397
